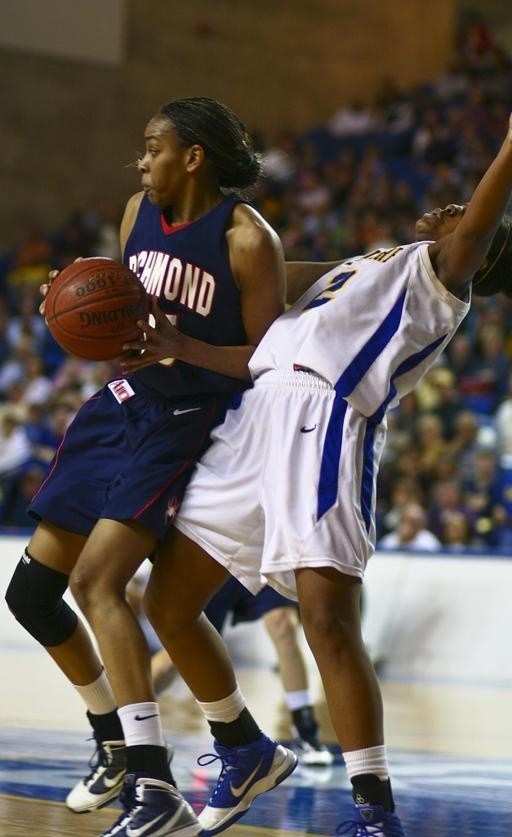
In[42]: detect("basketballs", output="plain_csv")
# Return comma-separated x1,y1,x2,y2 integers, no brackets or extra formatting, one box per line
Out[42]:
46,256,148,361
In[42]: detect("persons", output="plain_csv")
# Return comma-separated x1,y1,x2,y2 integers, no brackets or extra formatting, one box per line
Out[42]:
4,95,288,837
117,126,511,833
1,19,511,553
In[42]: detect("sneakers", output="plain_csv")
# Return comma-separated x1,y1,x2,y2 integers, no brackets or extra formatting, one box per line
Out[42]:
288,706,334,764
65,731,203,836
340,805,409,837
197,729,298,834
